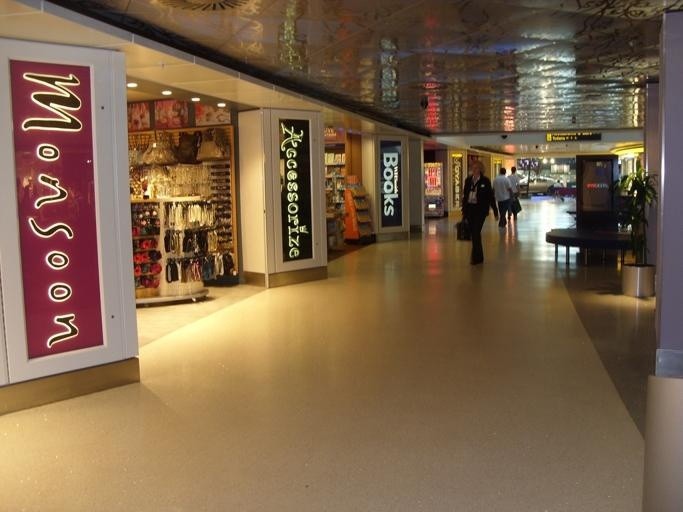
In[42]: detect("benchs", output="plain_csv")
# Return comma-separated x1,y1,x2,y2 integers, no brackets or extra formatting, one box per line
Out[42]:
546,229,634,266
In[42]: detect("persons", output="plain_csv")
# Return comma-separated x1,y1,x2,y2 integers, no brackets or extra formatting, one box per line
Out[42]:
461,160,499,265
554,180,564,202
492,167,514,227
507,167,521,220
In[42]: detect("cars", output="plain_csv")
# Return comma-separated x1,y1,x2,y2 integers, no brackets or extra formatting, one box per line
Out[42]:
516,177,554,195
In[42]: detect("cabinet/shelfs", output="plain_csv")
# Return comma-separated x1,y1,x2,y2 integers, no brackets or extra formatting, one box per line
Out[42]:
128,125,237,305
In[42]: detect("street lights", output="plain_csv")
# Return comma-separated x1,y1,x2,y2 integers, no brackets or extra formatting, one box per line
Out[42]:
520,160,539,197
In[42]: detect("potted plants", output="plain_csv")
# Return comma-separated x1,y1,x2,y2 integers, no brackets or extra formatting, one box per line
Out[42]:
608,165,656,297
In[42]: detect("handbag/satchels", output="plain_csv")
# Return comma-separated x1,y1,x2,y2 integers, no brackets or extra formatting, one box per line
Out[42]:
510,199,521,215
457,223,470,240
